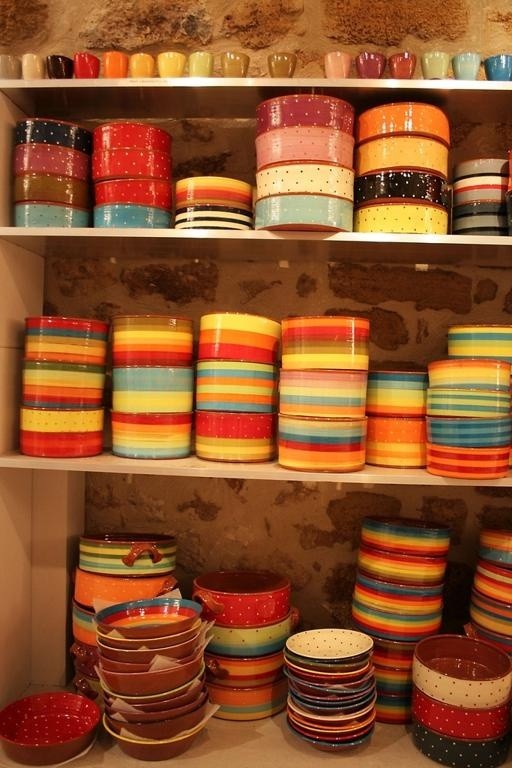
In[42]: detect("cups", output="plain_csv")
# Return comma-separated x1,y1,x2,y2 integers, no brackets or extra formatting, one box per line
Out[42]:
0,52,512,80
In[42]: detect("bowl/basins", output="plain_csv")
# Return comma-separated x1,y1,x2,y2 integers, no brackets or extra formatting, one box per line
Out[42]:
16,312,511,478
71,533,300,761
15,93,512,235
352,516,512,768
0,693,102,765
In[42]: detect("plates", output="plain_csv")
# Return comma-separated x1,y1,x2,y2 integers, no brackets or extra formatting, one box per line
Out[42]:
283,628,378,750
1,734,97,767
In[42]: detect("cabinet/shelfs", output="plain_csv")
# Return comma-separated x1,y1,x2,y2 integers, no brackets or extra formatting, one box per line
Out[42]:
0,77,512,768
